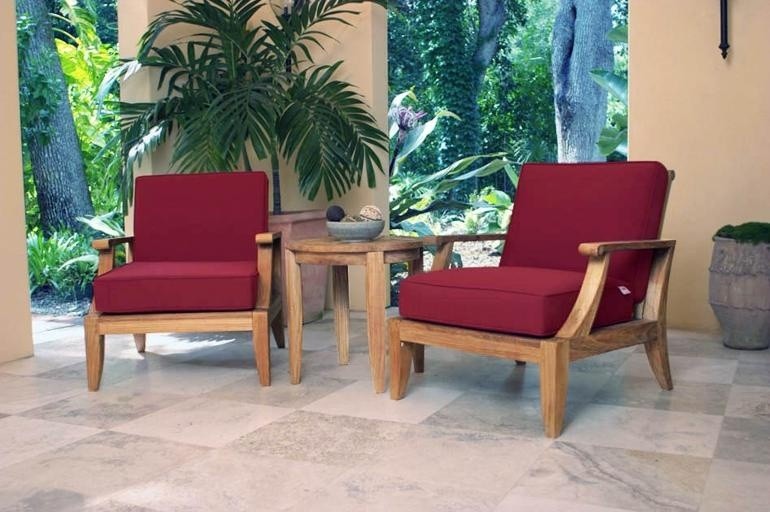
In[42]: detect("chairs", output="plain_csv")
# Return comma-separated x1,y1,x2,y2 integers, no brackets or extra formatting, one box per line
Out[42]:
85,173,285,391
389,171,676,440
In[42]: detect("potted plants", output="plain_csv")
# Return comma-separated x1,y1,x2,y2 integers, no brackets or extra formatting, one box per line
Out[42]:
96,0,393,324
709,221,770,351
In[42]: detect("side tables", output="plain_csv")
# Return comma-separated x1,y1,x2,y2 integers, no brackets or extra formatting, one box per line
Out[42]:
286,236,425,394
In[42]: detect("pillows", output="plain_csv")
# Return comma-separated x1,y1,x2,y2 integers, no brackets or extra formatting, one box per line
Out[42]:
499,162,669,299
132,173,268,260
